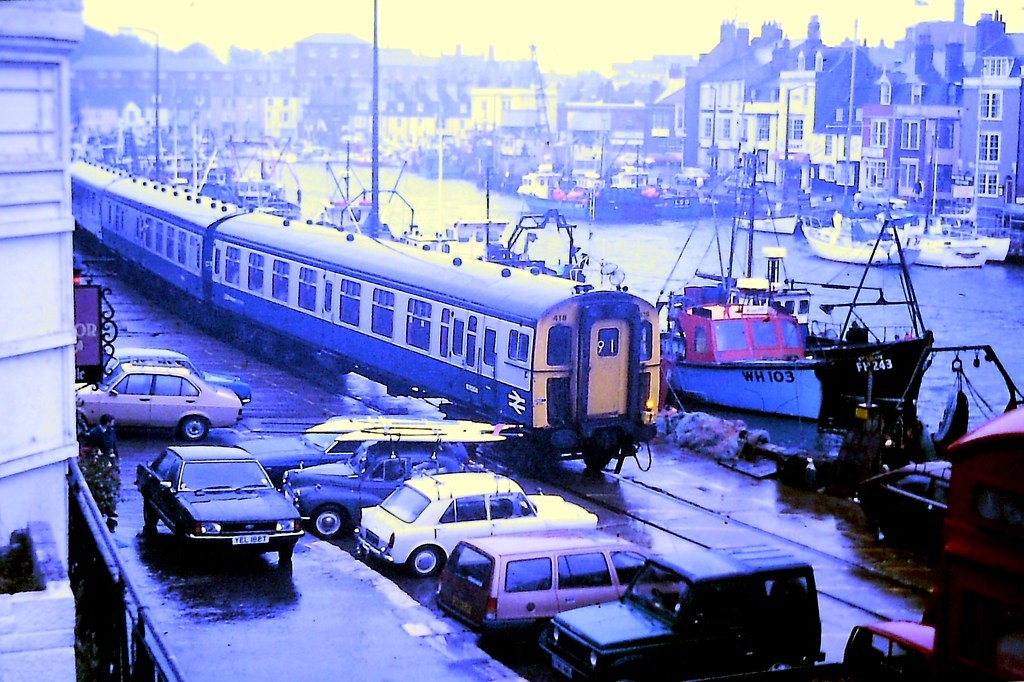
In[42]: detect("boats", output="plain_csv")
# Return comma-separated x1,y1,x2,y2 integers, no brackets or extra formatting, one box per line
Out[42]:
655,135,931,425
732,206,1011,267
517,119,702,222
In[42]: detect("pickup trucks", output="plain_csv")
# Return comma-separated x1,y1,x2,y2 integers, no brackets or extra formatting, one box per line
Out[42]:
809,621,937,682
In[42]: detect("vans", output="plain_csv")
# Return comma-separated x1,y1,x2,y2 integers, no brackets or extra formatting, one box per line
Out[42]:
434,537,660,637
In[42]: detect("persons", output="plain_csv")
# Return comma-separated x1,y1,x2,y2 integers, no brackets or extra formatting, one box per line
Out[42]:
89,414,121,517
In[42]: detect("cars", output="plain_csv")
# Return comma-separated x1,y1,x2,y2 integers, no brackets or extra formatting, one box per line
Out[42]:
358,473,597,582
280,436,472,539
138,446,304,560
723,174,762,195
853,187,907,212
76,362,242,444
860,461,953,533
77,349,253,410
673,167,710,187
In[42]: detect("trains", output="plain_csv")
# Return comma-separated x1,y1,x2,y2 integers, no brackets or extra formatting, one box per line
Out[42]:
72,163,662,472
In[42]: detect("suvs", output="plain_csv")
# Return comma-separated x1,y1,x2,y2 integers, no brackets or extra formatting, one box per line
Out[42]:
536,546,826,682
